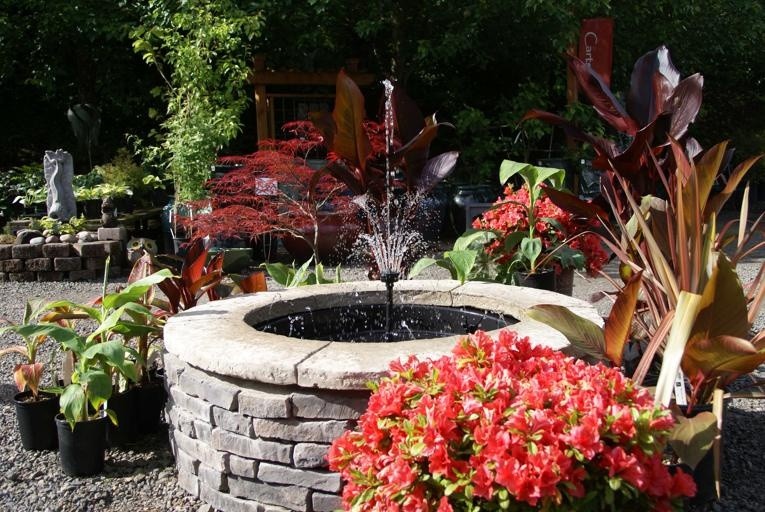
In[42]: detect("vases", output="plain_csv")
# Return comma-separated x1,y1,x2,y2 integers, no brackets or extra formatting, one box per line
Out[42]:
512,264,574,297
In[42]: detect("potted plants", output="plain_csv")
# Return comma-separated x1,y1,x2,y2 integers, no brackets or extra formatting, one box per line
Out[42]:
496,156,567,288
0,256,171,474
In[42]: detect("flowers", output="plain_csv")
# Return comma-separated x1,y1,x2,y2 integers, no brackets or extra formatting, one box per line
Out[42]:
466,177,603,275
